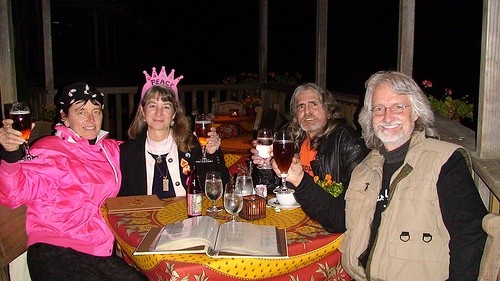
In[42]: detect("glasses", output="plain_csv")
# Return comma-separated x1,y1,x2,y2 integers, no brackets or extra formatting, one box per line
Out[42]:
370,103,413,116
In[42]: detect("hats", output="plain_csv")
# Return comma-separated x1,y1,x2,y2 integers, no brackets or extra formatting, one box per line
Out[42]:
60,82,103,112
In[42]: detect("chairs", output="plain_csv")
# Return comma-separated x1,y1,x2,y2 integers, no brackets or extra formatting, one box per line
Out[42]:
0,204,32,281
211,97,263,130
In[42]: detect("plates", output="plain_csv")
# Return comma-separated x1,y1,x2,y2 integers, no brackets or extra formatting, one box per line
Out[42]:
267,198,301,211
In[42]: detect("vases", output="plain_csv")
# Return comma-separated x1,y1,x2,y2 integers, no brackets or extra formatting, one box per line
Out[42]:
245,106,257,118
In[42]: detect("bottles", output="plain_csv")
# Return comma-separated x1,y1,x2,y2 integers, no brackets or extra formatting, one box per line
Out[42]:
186,162,202,218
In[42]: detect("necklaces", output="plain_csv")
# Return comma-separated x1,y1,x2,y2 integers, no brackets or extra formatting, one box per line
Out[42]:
150,130,169,163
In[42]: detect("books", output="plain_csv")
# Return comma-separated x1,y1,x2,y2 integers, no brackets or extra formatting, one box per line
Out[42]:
105,194,165,215
150,216,283,255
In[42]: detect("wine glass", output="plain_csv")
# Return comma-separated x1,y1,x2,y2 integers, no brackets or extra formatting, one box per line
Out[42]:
205,171,223,213
194,114,214,163
255,128,273,170
224,182,243,222
9,101,39,163
273,130,296,194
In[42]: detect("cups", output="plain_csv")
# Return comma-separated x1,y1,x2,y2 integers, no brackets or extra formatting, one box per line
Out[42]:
276,193,296,206
234,176,253,196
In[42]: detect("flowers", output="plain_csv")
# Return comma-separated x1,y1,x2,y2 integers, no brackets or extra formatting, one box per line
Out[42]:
41,101,57,121
313,173,344,198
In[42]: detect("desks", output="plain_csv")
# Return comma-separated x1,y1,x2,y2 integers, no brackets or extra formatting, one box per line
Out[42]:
196,136,256,178
101,192,356,281
213,114,247,135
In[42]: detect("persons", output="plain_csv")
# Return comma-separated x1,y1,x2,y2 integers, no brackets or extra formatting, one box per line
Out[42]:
250,83,372,198
119,66,231,200
0,82,150,281
271,71,489,281
